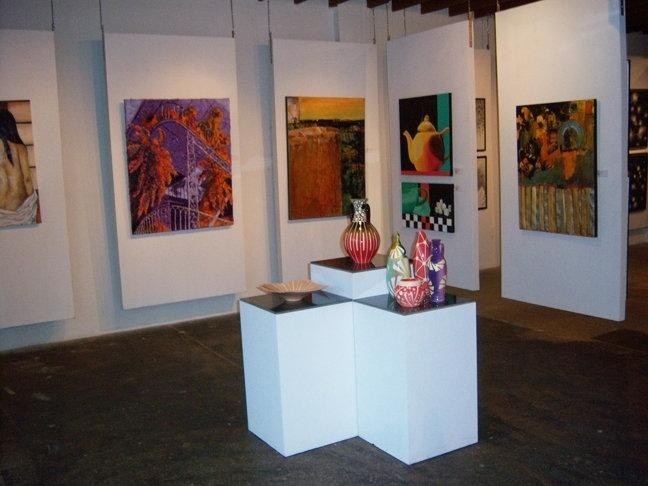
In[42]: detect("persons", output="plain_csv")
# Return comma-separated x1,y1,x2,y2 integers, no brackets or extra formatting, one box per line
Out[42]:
0,111,35,210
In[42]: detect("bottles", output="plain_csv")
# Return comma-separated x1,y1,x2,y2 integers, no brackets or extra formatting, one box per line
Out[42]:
388,229,447,309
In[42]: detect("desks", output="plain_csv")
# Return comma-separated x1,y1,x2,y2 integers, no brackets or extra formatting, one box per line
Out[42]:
239,253,478,465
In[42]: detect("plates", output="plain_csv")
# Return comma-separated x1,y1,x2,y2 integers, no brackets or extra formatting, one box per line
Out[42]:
257,280,328,303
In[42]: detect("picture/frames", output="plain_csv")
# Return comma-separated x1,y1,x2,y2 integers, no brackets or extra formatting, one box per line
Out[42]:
475,97,488,210
